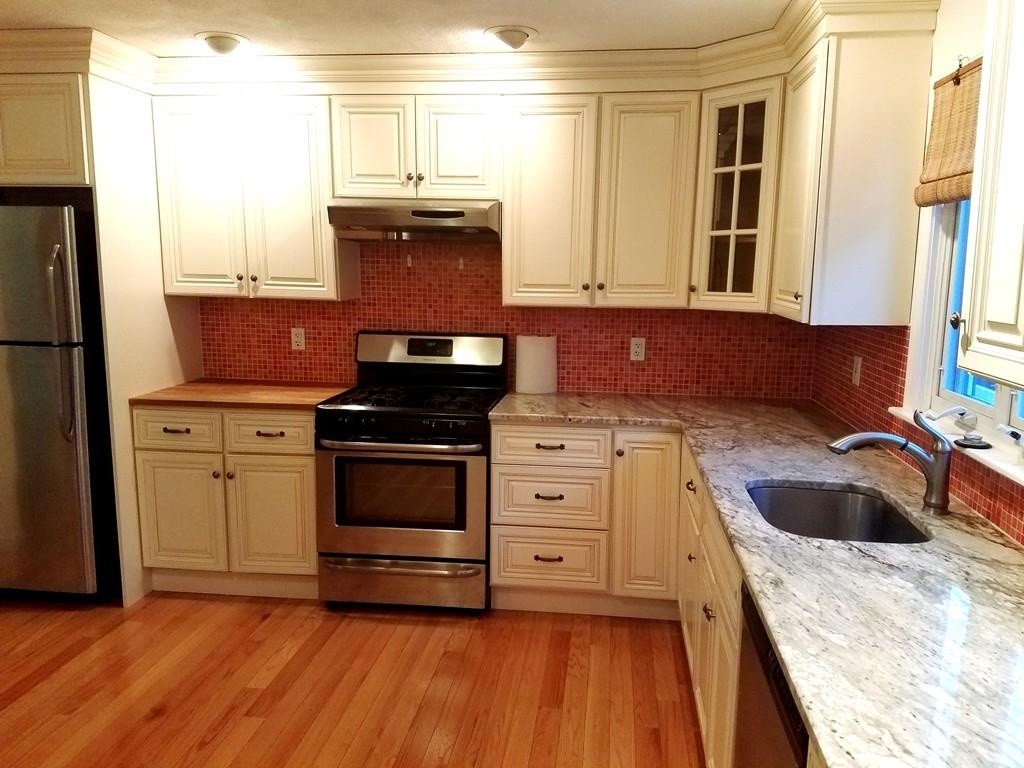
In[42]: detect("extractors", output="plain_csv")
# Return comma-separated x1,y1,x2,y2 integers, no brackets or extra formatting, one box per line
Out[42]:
327,199,502,240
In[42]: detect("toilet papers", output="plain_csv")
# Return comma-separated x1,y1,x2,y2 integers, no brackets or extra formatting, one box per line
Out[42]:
515,335,558,395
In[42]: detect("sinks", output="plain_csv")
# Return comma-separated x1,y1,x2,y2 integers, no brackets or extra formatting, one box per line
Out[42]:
744,478,936,545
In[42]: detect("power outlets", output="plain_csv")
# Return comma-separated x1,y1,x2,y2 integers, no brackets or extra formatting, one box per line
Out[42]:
850,354,864,386
631,337,647,359
291,327,305,350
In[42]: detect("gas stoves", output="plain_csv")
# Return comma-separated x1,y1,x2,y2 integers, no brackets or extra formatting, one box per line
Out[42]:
315,329,506,444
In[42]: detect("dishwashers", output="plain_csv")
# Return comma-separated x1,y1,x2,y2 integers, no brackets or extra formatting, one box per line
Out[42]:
732,579,810,768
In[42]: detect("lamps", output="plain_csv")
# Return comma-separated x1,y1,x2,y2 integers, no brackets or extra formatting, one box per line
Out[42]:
482,25,539,48
192,31,250,57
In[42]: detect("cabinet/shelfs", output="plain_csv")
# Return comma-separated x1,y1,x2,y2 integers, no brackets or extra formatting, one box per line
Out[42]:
491,431,613,584
154,92,332,298
677,452,746,768
133,410,316,572
692,74,784,313
332,92,495,197
762,42,829,325
502,93,704,305
611,429,679,601
0,73,90,184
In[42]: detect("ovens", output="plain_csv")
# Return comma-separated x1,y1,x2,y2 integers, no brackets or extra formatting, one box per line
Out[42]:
316,439,491,615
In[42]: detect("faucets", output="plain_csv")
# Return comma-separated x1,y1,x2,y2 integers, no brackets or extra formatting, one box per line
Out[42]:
824,408,952,516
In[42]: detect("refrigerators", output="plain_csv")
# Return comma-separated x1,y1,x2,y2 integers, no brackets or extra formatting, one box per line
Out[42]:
0,187,122,608
